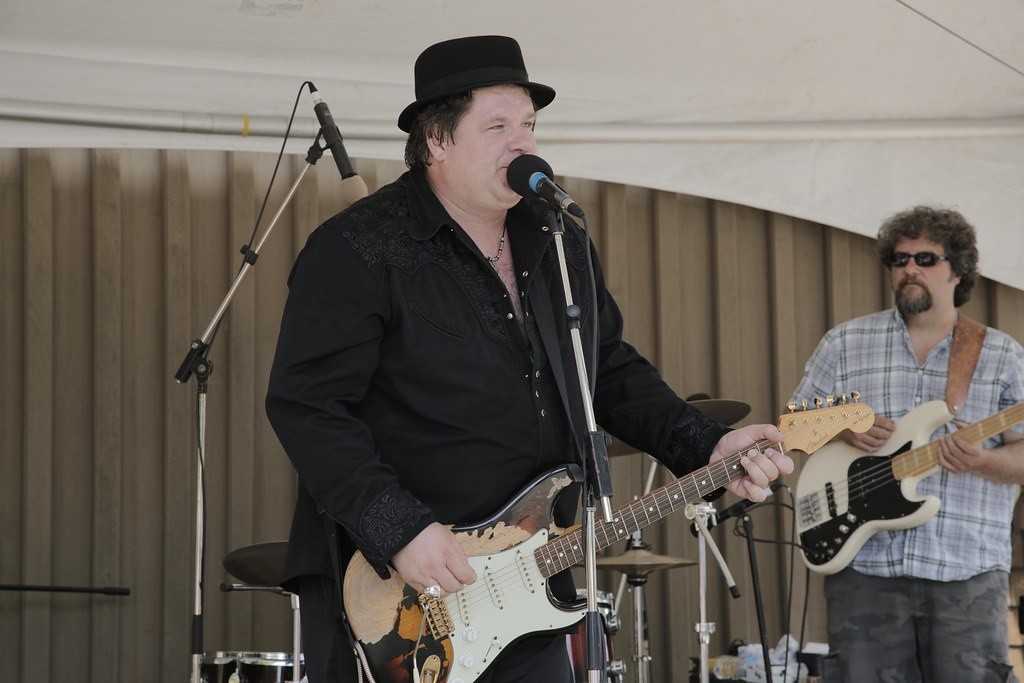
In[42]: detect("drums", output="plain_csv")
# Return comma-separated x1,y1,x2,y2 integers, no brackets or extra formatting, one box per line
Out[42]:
199,651,310,682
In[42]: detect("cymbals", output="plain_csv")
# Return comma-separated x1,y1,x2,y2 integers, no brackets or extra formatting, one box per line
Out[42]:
582,398,753,461
223,540,297,600
575,550,703,578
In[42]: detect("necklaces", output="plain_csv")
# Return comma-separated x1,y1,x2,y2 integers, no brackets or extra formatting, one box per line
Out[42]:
485,226,506,275
424,585,440,600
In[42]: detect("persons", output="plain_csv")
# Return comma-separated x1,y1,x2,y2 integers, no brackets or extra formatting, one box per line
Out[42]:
264,34,794,683
783,207,1024,683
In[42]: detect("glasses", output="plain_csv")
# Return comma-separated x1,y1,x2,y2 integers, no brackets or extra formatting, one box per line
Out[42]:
888,252,951,268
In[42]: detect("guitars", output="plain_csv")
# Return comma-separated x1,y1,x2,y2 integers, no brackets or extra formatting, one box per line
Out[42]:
795,399,1024,575
341,389,875,683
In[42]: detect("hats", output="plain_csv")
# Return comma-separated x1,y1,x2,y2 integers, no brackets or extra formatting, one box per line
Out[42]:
397,35,556,134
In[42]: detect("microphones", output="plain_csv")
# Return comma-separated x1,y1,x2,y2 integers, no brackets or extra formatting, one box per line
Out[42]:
507,155,585,218
691,484,783,538
308,81,368,206
